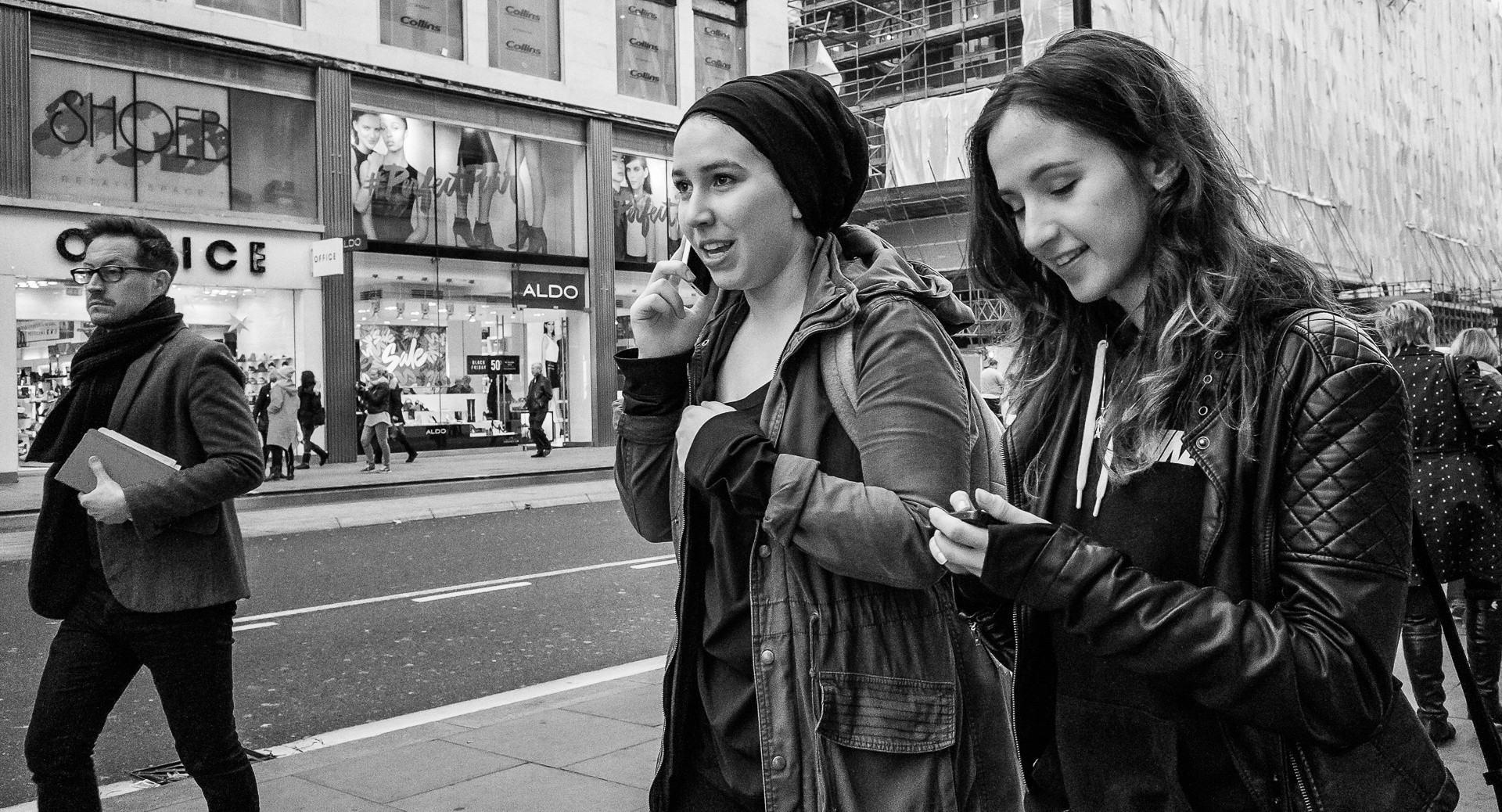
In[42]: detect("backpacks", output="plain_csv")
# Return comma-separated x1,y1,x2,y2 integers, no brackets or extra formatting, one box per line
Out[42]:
821,295,1008,510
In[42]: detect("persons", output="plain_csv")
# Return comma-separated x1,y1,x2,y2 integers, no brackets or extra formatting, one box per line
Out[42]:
522,363,553,457
453,127,504,250
350,110,431,245
1376,300,1502,747
447,375,517,440
355,368,419,473
611,151,656,265
24,214,265,812
930,28,1459,812
505,136,547,253
253,366,329,482
614,69,1024,812
541,321,559,417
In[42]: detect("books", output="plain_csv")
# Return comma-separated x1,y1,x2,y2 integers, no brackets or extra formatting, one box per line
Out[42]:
54,428,182,522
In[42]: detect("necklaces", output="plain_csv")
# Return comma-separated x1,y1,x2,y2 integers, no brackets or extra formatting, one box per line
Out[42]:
1094,278,1151,438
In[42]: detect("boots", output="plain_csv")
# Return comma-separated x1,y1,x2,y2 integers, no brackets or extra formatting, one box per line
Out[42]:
1402,614,1456,749
405,446,417,462
474,217,504,250
287,465,294,480
263,466,281,481
297,454,310,469
508,220,530,249
526,226,547,254
316,449,329,466
453,213,482,247
1465,610,1502,726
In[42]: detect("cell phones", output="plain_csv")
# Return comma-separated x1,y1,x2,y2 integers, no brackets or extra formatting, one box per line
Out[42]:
681,239,712,298
948,509,1006,528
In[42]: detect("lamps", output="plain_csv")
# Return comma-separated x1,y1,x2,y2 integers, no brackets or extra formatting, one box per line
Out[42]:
371,274,380,316
469,280,476,322
422,277,429,319
446,278,454,320
397,276,404,320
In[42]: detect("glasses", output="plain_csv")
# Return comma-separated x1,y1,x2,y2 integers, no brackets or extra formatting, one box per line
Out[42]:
70,265,161,284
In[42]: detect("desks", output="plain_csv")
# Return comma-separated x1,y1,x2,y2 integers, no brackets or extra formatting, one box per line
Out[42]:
512,411,554,444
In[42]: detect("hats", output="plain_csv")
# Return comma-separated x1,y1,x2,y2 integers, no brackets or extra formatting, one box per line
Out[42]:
277,366,296,380
675,69,869,236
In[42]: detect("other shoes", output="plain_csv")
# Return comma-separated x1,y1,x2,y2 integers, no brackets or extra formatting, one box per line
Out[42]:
531,448,543,457
361,463,375,472
544,449,550,456
381,464,390,471
